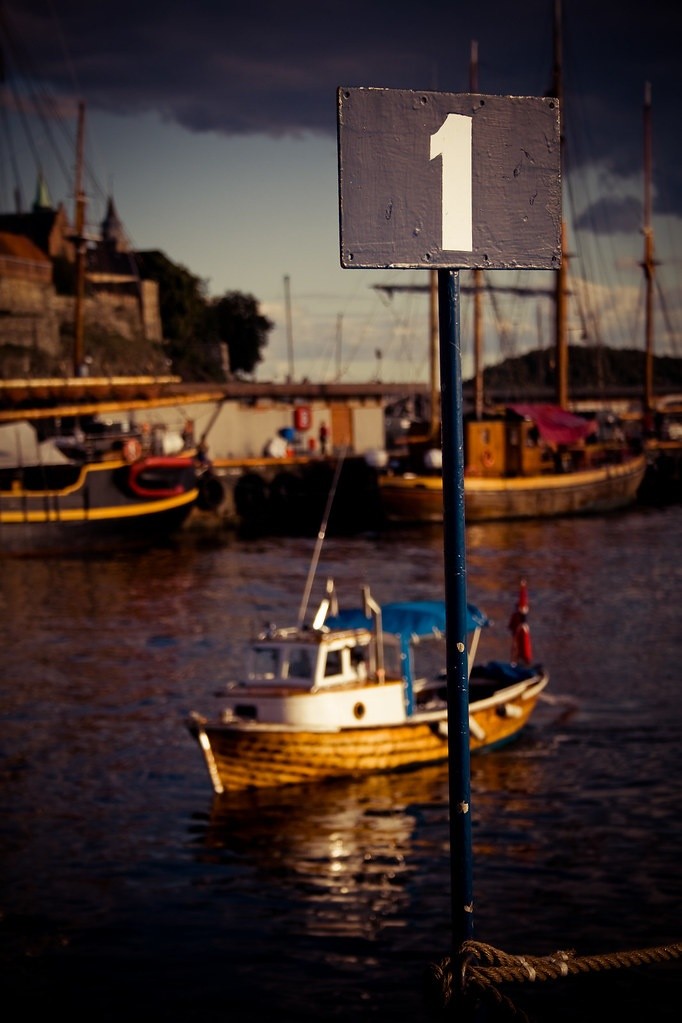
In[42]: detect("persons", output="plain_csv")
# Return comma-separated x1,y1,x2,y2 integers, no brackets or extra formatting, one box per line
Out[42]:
319,422,328,456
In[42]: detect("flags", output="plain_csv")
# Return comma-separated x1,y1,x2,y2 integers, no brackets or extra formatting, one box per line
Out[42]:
508,579,535,662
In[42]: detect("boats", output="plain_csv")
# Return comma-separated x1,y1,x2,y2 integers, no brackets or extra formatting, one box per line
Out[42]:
368,84,681,528
0,424,208,559
179,576,551,796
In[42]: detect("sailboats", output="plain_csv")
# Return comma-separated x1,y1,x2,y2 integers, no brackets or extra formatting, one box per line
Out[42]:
1,99,225,457
